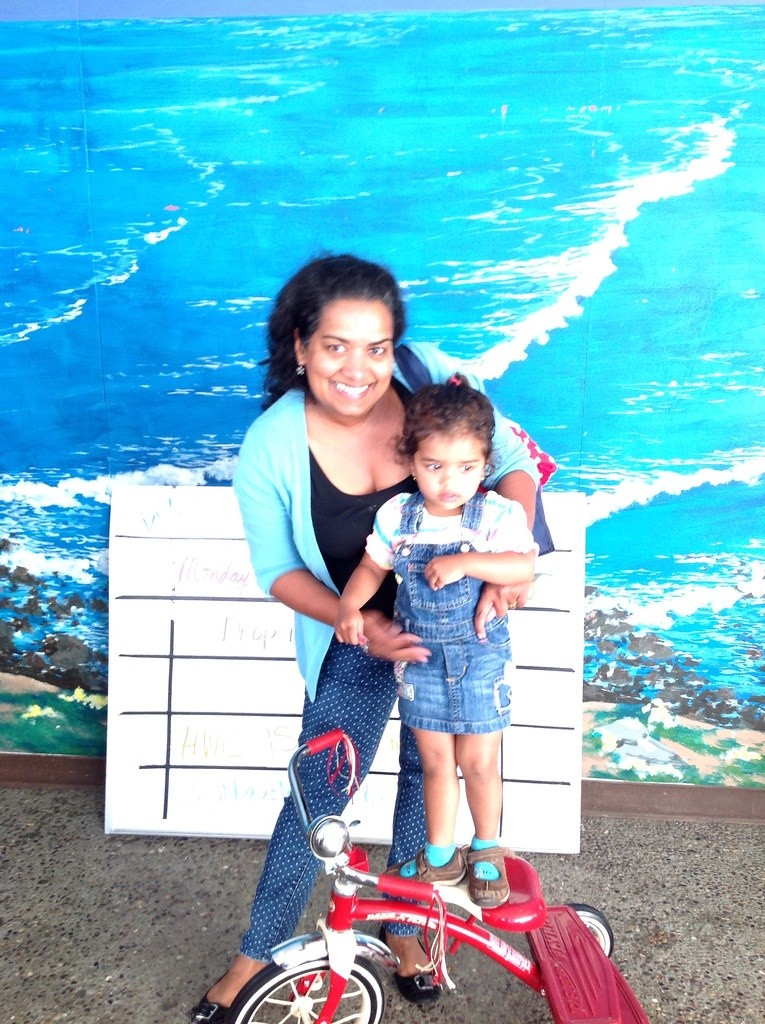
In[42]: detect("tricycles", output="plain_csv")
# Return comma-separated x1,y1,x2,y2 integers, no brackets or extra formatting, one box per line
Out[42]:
225,732,649,1023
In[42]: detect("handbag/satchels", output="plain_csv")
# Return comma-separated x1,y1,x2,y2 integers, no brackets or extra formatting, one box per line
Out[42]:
394,346,554,556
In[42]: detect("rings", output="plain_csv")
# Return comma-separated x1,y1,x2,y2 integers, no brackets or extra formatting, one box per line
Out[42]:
509,602,517,607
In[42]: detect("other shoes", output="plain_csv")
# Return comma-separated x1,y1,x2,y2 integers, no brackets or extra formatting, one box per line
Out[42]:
467,844,515,909
378,925,441,1003
189,957,282,1024
383,846,466,886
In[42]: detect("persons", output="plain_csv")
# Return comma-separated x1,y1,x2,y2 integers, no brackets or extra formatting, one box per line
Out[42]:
185,256,539,1024
335,375,539,909
